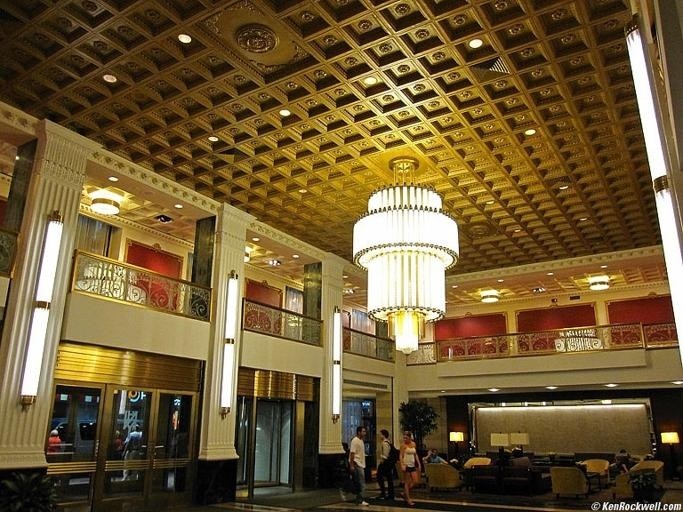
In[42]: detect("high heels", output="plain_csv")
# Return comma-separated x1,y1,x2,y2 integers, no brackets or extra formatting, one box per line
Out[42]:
404,497,416,506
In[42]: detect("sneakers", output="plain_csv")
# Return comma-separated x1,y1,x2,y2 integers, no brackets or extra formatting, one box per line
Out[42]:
338,487,348,502
375,493,386,499
356,501,370,507
383,495,395,501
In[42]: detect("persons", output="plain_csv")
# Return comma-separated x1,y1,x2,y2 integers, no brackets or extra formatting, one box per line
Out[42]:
616,449,632,473
339,427,370,505
47,429,62,452
115,430,123,449
120,424,143,481
376,430,400,500
398,430,422,506
424,449,449,465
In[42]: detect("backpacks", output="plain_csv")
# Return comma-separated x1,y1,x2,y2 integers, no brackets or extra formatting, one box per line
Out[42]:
383,440,401,464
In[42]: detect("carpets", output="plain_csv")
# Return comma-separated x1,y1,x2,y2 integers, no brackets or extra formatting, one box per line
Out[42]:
230,486,382,511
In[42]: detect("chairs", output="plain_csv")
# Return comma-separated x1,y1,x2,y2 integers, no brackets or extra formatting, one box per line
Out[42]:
418,450,666,504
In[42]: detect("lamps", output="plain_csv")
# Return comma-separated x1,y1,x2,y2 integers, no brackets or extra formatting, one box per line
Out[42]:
216,269,240,420
88,198,120,216
330,304,342,425
17,208,64,410
659,429,679,478
349,155,462,355
589,279,609,290
448,429,464,456
619,12,682,379
244,249,250,262
478,294,498,303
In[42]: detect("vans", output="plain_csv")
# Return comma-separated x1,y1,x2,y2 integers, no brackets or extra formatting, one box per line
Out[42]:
46,419,144,462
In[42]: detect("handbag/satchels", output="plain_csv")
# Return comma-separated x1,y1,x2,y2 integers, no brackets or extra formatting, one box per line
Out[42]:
346,469,363,495
